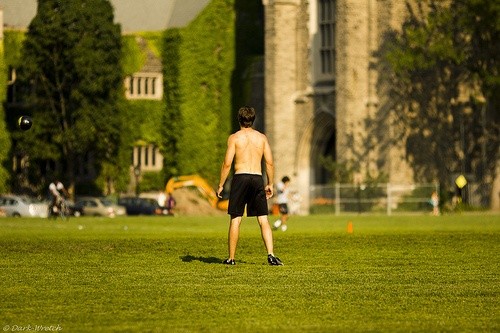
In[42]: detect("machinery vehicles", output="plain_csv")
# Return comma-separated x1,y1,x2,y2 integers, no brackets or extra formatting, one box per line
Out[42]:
165,174,247,214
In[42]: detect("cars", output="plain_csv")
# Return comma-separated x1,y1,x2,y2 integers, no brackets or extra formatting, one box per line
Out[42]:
78,197,126,218
0,194,49,218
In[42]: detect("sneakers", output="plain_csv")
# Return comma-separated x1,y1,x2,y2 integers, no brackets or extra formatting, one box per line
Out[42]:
223,258,236,265
267,254,282,265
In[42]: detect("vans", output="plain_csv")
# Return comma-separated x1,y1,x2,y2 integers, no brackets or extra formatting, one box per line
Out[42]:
120,196,169,216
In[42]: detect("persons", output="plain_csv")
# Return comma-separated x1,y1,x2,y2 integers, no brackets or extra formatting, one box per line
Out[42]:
49,177,68,215
216,106,284,266
272,176,290,232
